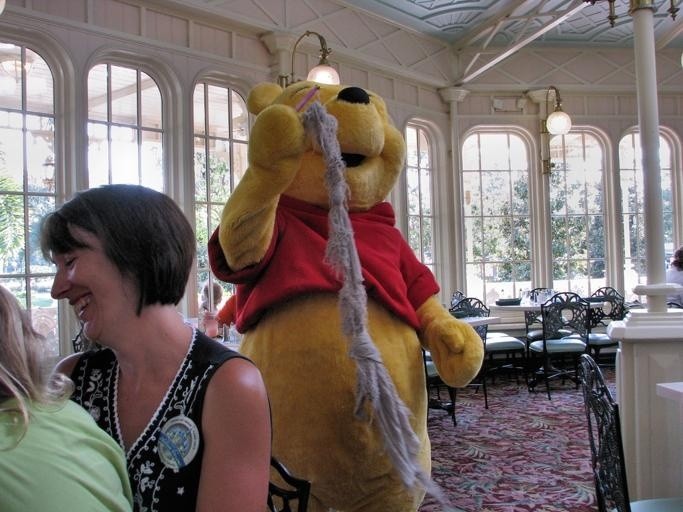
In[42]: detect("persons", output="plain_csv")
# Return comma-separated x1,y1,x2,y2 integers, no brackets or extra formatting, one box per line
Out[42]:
196,283,223,315
665,244,683,309
1,285,134,511
40,184,274,512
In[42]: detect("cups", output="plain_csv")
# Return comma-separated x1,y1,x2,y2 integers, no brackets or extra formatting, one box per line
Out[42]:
203,313,218,340
183,317,199,328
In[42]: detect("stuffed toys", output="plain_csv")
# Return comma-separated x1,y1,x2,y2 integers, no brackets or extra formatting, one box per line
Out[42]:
207,81,485,510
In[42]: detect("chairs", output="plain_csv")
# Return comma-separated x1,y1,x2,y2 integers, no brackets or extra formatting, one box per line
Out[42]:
577,353,681,512
413,285,681,428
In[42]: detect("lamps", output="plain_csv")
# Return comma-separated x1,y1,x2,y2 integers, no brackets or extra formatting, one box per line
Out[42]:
543,85,574,136
290,32,341,89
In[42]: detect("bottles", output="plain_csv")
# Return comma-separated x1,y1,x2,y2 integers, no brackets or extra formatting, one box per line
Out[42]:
221,322,238,345
451,297,459,312
517,288,558,307
